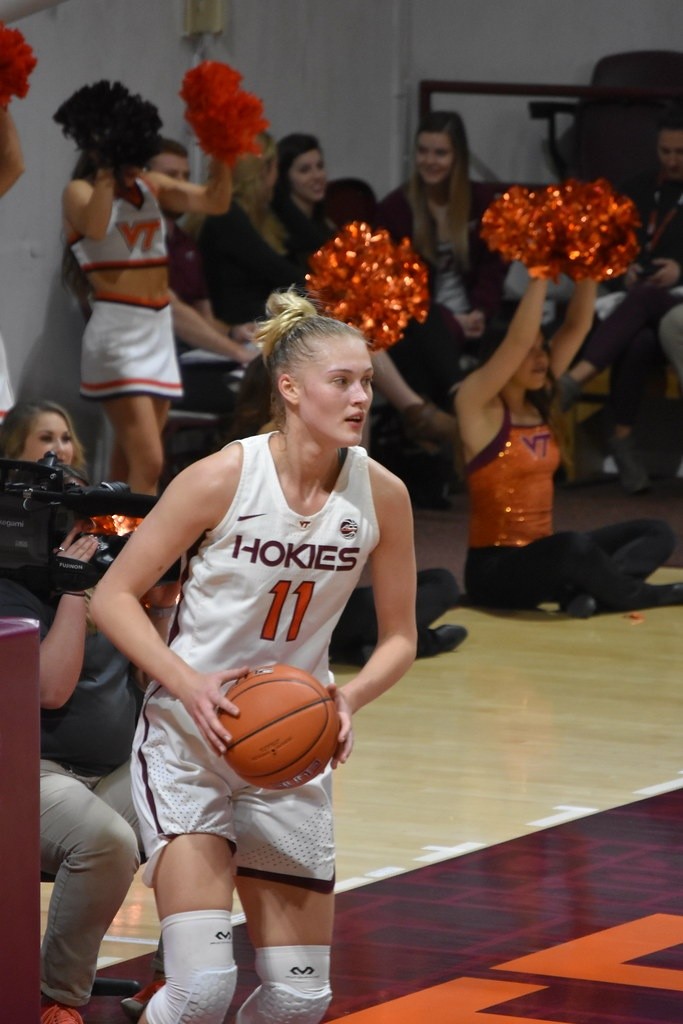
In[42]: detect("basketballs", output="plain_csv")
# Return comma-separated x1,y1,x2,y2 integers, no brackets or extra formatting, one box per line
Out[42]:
216,664,341,792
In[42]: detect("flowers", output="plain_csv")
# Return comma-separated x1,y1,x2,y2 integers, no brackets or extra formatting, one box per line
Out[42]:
0,18,644,356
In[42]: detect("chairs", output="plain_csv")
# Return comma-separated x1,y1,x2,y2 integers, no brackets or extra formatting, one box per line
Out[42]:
57,265,253,472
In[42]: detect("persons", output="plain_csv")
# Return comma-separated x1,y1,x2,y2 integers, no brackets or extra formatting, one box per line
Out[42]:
0,107,683,668
89,289,418,1024
0,403,163,1024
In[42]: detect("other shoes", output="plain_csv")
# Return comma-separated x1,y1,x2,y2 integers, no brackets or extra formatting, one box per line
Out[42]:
410,493,453,509
556,375,580,412
610,582,683,612
559,584,597,619
436,624,468,654
352,644,375,666
609,436,650,493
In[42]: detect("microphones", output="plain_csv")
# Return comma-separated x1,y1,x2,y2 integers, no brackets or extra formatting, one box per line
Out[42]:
57,490,159,517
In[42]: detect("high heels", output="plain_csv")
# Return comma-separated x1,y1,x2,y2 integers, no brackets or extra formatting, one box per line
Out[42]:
402,402,460,455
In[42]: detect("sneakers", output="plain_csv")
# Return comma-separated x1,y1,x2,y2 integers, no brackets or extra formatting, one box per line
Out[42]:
120,979,167,1020
40,1000,84,1024
